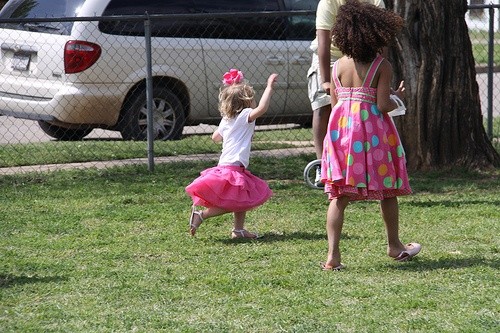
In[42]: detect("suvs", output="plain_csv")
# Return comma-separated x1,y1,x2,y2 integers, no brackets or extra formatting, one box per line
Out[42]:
0,0,323,143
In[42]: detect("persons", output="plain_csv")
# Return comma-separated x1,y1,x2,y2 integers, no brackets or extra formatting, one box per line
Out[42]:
306,0,385,186
184,68,279,240
320,1,422,269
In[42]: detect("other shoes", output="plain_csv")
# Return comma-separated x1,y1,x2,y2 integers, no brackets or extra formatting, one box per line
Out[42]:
315,169,324,187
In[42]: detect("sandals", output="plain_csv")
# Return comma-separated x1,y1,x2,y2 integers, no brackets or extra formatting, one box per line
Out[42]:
189,204,203,235
323,263,346,271
393,243,421,261
232,229,247,238
